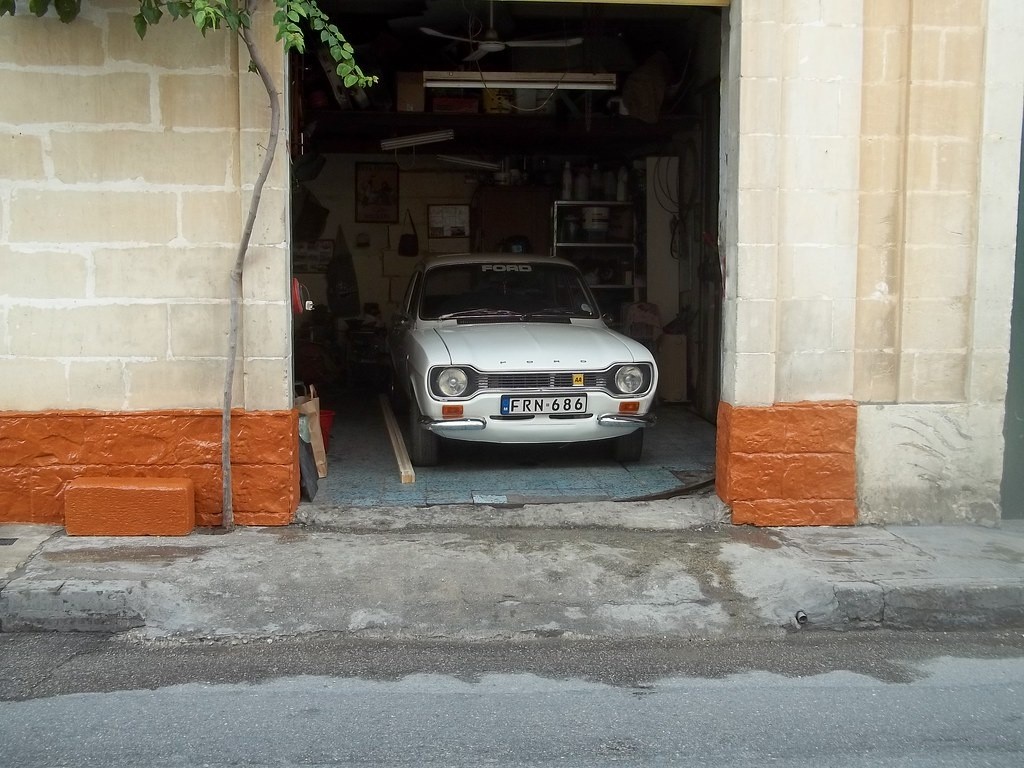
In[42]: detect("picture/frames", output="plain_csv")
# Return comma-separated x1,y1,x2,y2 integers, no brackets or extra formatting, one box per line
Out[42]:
292,238,335,275
426,203,470,239
355,161,400,224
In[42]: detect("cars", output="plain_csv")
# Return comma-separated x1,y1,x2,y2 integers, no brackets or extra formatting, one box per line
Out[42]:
392,252,659,467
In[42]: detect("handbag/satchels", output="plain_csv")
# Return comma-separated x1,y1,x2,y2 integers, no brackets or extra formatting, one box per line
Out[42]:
398,207,419,257
296,411,320,502
294,381,329,479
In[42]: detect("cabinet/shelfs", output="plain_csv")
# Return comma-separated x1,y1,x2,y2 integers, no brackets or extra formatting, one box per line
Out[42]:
550,199,638,328
630,155,680,330
470,186,554,257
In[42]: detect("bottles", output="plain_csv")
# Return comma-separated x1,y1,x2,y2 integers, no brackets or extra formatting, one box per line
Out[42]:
561,161,629,201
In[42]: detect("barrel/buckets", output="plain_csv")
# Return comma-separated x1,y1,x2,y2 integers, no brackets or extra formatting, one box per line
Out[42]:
319,410,335,454
580,207,609,232
492,172,512,185
480,88,556,114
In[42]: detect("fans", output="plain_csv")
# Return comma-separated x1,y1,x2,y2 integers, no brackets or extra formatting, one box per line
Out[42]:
419,0,584,111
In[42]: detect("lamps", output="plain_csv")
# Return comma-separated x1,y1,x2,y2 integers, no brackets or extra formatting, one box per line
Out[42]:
422,70,617,91
381,129,453,151
434,153,501,171
